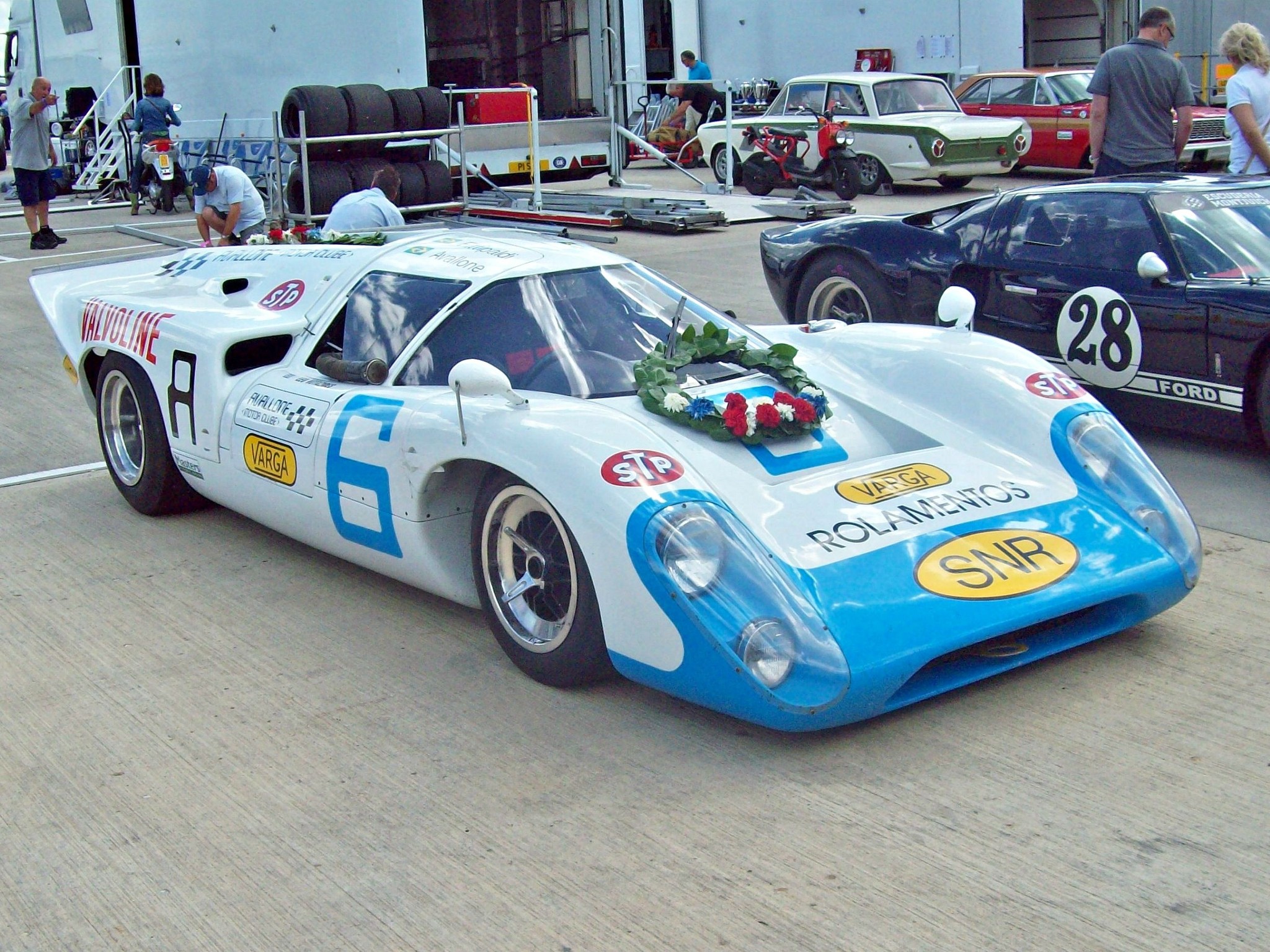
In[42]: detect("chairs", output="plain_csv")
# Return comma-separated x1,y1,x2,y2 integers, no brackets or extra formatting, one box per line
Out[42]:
172,136,290,211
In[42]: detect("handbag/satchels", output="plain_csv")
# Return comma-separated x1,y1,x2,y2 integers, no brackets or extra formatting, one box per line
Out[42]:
165,117,170,127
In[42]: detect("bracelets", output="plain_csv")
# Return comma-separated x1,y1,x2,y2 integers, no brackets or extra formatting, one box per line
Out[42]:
41,96,48,109
667,116,671,122
1088,155,1100,163
221,234,229,239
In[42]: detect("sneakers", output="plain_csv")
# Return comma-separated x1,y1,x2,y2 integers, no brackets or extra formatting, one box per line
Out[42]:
30,233,58,250
39,225,67,244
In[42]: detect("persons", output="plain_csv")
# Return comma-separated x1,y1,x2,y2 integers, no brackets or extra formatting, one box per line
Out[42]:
661,78,726,167
9,77,66,250
681,50,713,89
1086,6,1197,176
129,73,195,216
1219,22,1270,175
320,164,404,231
191,164,267,247
122,112,130,119
0,94,11,150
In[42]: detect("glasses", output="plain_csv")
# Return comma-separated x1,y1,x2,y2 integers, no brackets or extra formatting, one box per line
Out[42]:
1160,23,1175,41
681,58,688,61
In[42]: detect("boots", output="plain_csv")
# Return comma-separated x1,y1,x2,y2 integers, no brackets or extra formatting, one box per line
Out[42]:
184,184,195,211
128,192,140,215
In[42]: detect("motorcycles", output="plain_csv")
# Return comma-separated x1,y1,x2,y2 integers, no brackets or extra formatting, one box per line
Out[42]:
123,101,186,214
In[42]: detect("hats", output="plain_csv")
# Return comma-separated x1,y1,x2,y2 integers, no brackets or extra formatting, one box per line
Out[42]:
191,165,212,196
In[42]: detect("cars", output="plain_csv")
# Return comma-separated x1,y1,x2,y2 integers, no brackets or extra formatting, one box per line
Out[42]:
702,67,1030,191
941,70,1187,178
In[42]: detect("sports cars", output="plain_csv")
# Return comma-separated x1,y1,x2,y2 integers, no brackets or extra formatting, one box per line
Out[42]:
757,169,1270,454
30,220,1205,728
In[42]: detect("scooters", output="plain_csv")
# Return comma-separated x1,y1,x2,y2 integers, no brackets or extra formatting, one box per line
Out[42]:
736,102,861,200
